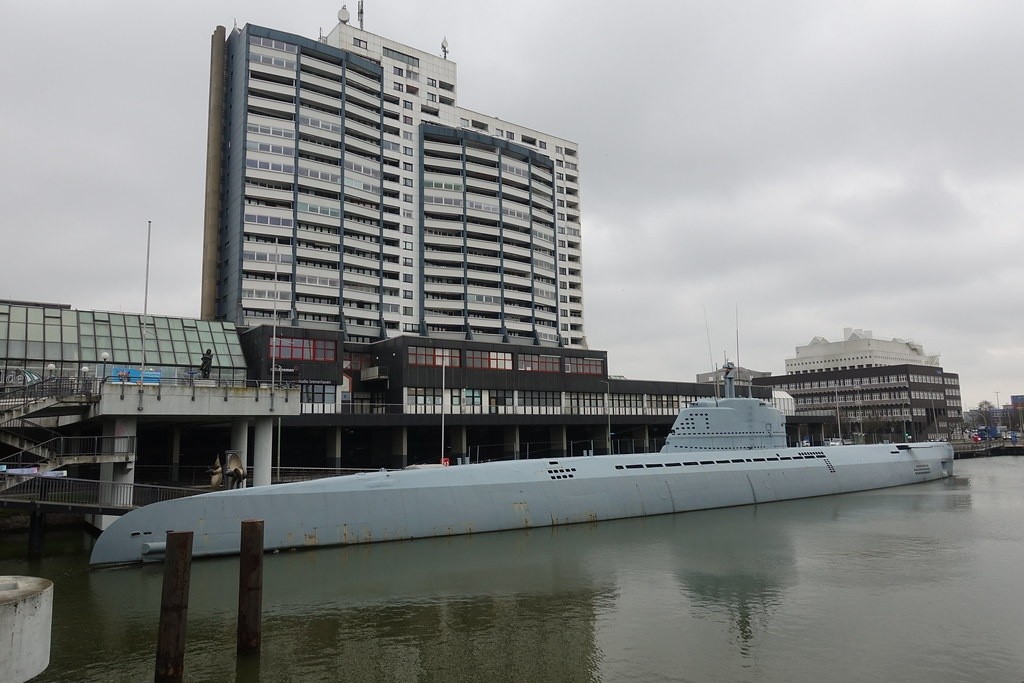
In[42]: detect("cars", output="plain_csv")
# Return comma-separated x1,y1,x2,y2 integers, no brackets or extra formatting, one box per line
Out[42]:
798,421,1024,447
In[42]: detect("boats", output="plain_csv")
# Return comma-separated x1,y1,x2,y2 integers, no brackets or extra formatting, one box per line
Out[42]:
90,308,955,569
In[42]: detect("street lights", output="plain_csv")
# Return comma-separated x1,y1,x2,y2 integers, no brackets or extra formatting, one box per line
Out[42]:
45,351,110,395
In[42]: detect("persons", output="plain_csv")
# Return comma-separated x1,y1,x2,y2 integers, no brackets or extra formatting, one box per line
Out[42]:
199,349,213,378
